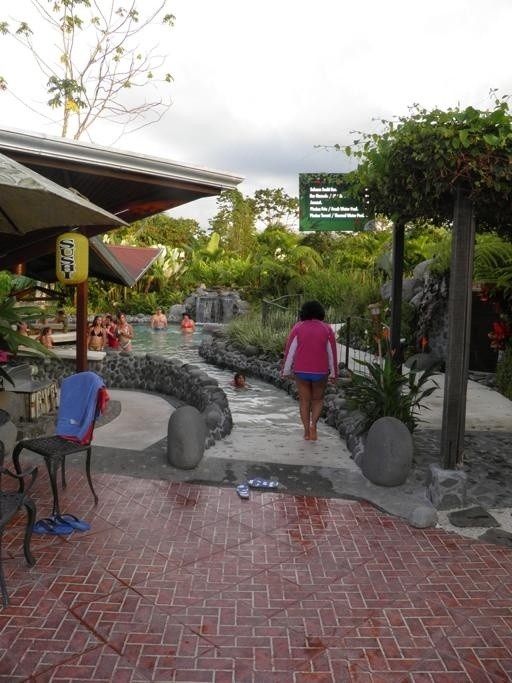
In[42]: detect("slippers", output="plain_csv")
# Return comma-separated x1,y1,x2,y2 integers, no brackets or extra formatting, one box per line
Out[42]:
32,512,91,535
237,478,279,498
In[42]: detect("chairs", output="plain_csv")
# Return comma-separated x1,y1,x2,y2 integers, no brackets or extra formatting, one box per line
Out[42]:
0,439,38,608
12,371,105,521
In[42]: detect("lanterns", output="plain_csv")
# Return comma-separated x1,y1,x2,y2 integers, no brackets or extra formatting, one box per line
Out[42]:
51,231,90,287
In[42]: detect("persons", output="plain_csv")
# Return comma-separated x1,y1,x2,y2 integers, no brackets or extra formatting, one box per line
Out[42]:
19,307,117,351
180,313,197,334
281,299,337,441
112,311,136,351
225,371,252,390
152,307,168,331
